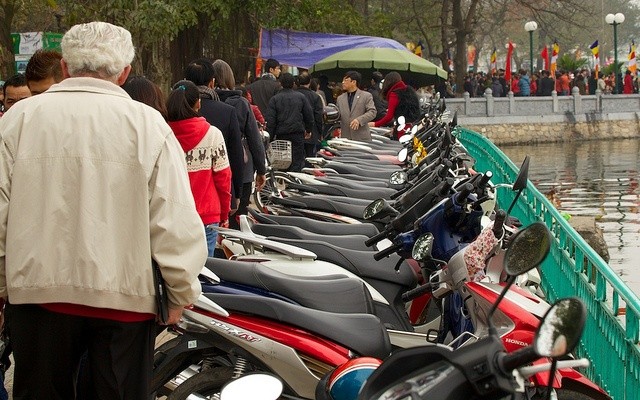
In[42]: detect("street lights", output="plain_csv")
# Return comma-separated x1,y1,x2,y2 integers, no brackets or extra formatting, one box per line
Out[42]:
505,42,516,94
605,13,625,95
524,21,538,82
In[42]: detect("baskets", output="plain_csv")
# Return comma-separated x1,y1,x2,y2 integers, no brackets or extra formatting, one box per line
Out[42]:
266,139,293,170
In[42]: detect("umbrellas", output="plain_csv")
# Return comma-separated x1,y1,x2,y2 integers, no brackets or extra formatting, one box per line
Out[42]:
308,48,449,80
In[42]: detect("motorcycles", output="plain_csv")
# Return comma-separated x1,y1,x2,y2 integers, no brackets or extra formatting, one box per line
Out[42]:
146,197,610,400
290,86,531,198
241,120,300,215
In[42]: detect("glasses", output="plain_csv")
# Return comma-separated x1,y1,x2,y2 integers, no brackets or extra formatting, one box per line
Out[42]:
275,67,281,70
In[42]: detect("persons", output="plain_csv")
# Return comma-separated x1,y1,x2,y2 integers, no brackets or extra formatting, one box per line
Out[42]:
337,72,377,143
211,59,266,213
241,56,334,168
182,55,241,215
165,82,231,258
27,51,64,96
368,72,422,140
368,70,387,121
0,72,31,111
128,77,164,115
0,22,209,400
444,65,640,96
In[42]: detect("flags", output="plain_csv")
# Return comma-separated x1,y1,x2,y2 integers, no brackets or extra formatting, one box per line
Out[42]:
541,44,549,72
590,39,599,80
627,38,637,78
414,45,422,57
491,46,497,63
550,38,558,78
505,42,515,84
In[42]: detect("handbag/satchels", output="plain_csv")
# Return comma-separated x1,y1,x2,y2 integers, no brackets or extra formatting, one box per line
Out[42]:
153,261,170,325
